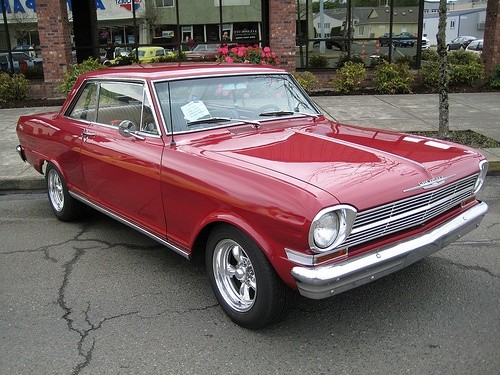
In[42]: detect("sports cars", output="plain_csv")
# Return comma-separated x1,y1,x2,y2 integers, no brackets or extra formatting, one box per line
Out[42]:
16,61,491,332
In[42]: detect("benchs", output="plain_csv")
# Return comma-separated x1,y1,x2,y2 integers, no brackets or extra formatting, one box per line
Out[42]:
74,102,186,132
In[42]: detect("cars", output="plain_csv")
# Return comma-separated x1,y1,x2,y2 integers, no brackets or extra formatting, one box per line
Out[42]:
0,44,45,77
446,36,479,52
421,34,430,51
378,32,416,48
464,39,484,56
99,46,192,67
184,43,239,61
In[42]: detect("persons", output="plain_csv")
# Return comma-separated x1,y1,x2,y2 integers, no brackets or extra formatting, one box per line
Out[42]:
421,34,430,51
105,38,115,60
29,44,36,58
222,31,230,41
